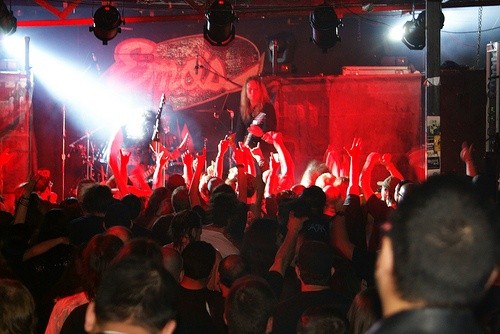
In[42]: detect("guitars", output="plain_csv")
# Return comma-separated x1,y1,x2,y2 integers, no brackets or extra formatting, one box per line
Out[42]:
240,111,268,148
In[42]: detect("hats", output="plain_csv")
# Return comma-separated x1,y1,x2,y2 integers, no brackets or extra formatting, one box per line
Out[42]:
182,240,216,280
297,241,333,285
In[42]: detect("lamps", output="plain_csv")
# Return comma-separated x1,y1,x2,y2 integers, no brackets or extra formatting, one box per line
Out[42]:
88,0,126,46
308,0,344,53
404,0,445,51
202,0,238,47
0,0,18,35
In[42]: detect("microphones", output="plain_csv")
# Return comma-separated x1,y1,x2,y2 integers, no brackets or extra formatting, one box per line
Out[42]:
67,144,74,158
197,55,198,75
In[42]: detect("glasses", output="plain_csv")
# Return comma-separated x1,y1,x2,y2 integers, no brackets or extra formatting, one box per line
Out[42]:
364,222,396,259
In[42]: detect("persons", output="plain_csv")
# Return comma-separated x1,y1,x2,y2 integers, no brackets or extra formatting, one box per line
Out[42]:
234,76,277,204
0,131,476,334
108,109,162,189
366,173,500,334
